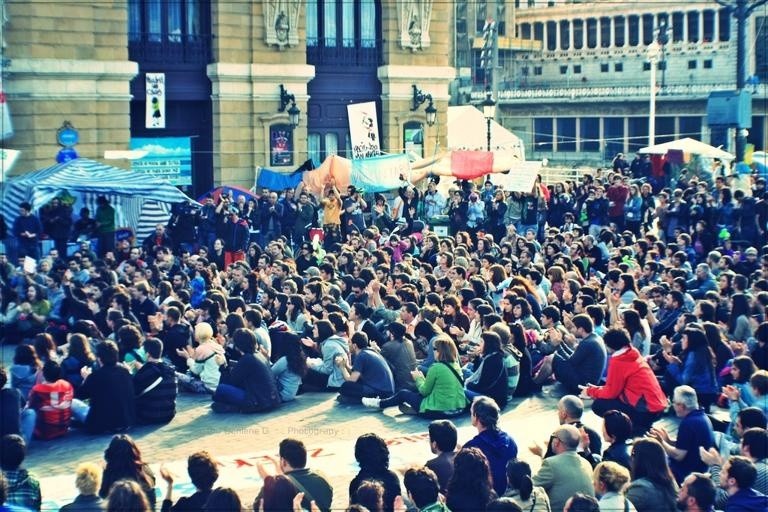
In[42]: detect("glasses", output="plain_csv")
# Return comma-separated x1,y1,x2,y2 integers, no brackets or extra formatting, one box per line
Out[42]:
433,347,437,350
351,290,360,294
286,302,292,305
550,435,558,440
302,247,307,250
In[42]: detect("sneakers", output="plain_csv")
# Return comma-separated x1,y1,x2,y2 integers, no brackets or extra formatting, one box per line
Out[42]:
399,403,417,415
361,396,382,411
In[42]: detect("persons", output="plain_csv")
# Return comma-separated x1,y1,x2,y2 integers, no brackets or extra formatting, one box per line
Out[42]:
0,149,768,512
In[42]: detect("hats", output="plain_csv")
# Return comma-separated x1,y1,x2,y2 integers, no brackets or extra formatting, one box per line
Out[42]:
395,217,407,225
384,322,406,336
336,274,354,285
351,188,363,193
453,190,463,196
304,265,320,277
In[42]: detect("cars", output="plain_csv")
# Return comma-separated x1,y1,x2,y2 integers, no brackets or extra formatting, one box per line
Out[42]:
737,150,768,196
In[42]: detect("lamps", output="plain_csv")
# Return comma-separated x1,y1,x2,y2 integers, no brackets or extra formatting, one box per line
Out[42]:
278,85,300,129
410,85,437,127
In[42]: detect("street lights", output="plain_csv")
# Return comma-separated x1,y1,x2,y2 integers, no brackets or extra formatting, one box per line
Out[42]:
645,41,661,148
483,94,494,184
